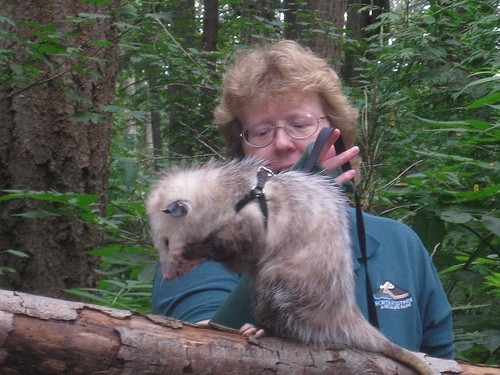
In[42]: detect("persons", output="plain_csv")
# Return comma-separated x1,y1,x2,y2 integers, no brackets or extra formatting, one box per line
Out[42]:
153,39,455,360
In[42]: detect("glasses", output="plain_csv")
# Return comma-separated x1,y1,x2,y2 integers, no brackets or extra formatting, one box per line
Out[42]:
238,110,329,148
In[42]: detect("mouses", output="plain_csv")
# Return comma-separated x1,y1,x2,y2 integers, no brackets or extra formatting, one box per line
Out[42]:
145,156,441,375
